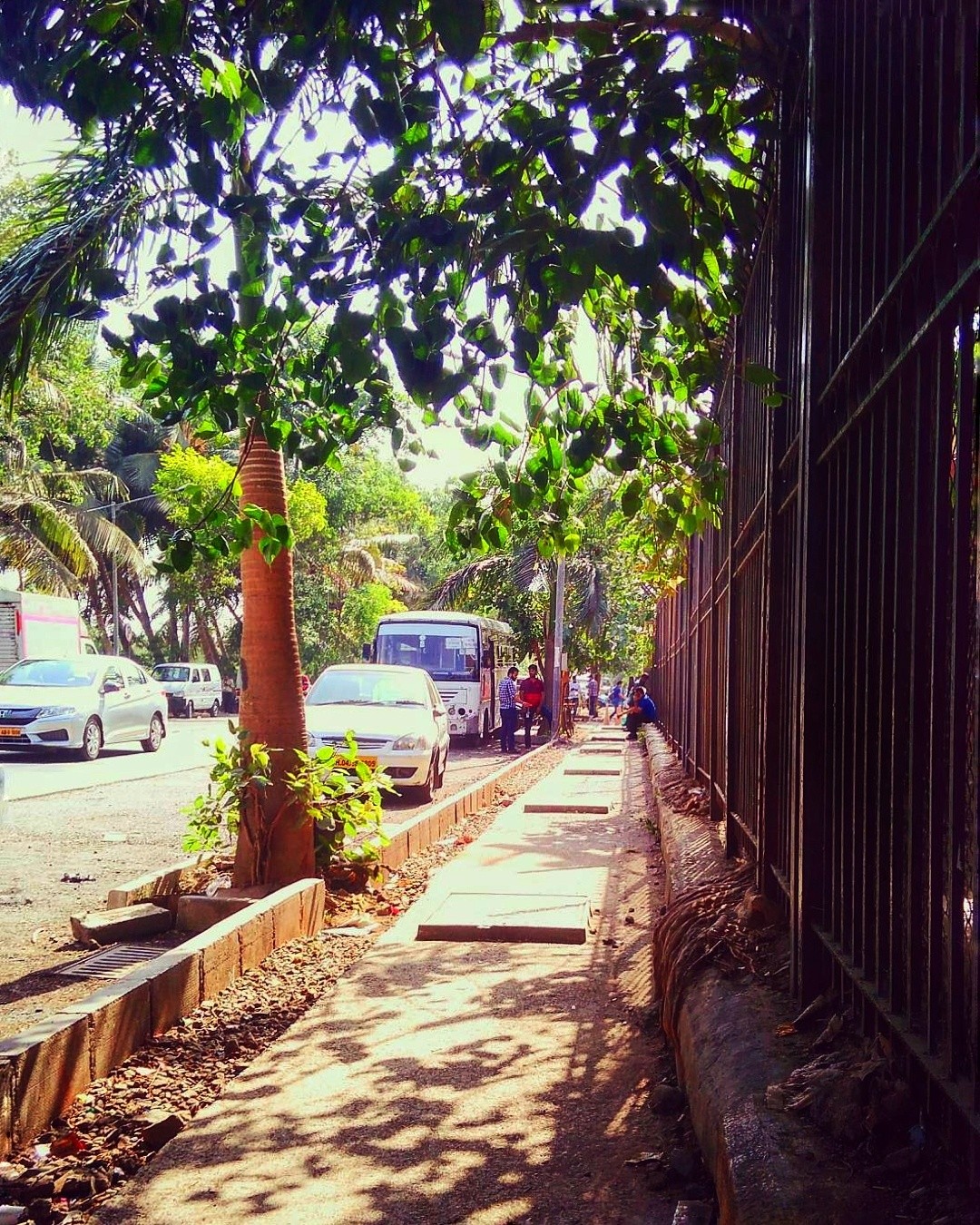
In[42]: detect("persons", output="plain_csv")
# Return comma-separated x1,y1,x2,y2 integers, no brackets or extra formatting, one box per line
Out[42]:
609,680,657,740
586,674,598,715
498,664,552,754
569,675,580,715
221,672,236,713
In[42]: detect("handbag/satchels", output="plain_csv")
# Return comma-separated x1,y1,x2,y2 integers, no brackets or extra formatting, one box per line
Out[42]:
609,692,616,700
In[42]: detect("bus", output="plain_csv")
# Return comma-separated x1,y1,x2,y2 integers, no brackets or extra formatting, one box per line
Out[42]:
361,610,515,747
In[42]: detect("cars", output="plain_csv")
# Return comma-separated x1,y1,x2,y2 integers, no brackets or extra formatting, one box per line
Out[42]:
601,677,612,697
0,654,169,762
303,663,451,802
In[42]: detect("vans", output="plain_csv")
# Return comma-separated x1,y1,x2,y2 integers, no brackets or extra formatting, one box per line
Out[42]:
151,662,223,718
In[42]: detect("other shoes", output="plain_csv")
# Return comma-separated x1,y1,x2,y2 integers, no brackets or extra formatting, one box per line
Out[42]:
626,733,637,741
501,749,509,753
508,749,521,754
